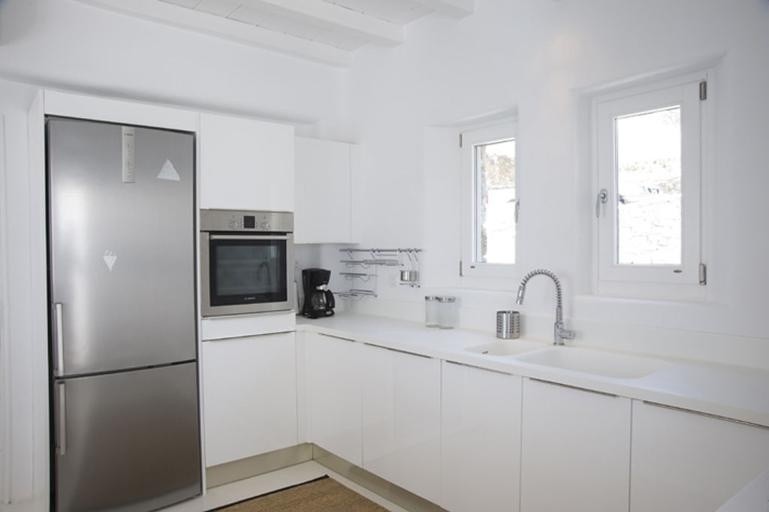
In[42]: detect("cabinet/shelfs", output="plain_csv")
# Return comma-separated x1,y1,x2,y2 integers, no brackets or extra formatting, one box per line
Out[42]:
198,110,296,214
329,244,420,297
629,393,747,511
308,332,440,512
293,136,352,244
194,332,300,510
444,354,632,512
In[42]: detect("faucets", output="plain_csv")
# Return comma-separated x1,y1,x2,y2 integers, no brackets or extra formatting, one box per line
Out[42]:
515,267,577,345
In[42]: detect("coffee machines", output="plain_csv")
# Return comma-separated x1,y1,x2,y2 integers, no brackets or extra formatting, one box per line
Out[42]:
301,269,335,319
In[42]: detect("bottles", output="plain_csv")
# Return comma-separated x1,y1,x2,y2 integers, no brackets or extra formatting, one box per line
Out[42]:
435,295,457,330
424,295,439,327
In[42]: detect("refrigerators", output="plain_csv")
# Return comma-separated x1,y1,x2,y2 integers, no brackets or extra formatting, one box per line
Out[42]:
45,117,203,512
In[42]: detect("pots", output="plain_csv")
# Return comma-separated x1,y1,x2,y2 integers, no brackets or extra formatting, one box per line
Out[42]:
400,270,417,281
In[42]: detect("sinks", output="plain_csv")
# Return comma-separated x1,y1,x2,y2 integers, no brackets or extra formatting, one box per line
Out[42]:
451,333,543,359
510,347,657,380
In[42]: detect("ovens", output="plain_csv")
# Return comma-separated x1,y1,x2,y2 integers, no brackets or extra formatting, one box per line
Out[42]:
199,208,295,317
